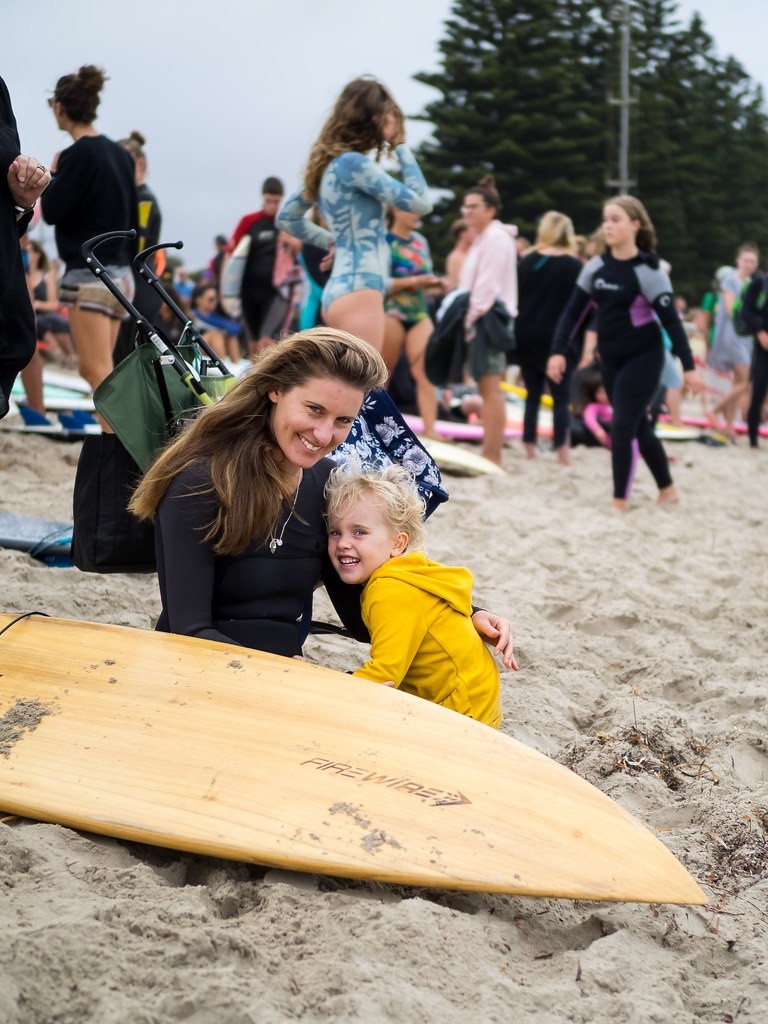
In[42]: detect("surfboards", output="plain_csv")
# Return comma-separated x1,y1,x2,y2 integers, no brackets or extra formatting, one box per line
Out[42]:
396,405,557,443
9,367,98,413
3,421,510,480
0,592,721,909
218,233,253,319
656,423,727,446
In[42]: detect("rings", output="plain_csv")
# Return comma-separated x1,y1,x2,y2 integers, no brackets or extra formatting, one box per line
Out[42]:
38,165,46,172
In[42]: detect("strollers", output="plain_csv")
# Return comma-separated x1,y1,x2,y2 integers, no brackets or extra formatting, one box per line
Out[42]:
81,230,451,638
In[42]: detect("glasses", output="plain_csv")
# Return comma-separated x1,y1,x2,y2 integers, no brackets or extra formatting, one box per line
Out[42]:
46,95,59,108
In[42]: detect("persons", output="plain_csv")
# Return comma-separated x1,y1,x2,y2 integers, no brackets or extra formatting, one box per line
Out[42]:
324,463,504,730
0,62,768,507
128,325,519,687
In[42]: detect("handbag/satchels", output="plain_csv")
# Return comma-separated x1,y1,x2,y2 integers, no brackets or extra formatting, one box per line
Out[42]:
70,432,156,574
332,386,449,525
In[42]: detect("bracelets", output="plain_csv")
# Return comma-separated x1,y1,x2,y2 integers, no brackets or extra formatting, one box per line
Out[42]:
14,202,35,214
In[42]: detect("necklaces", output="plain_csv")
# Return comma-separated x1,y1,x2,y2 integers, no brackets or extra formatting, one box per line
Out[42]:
268,468,301,553
